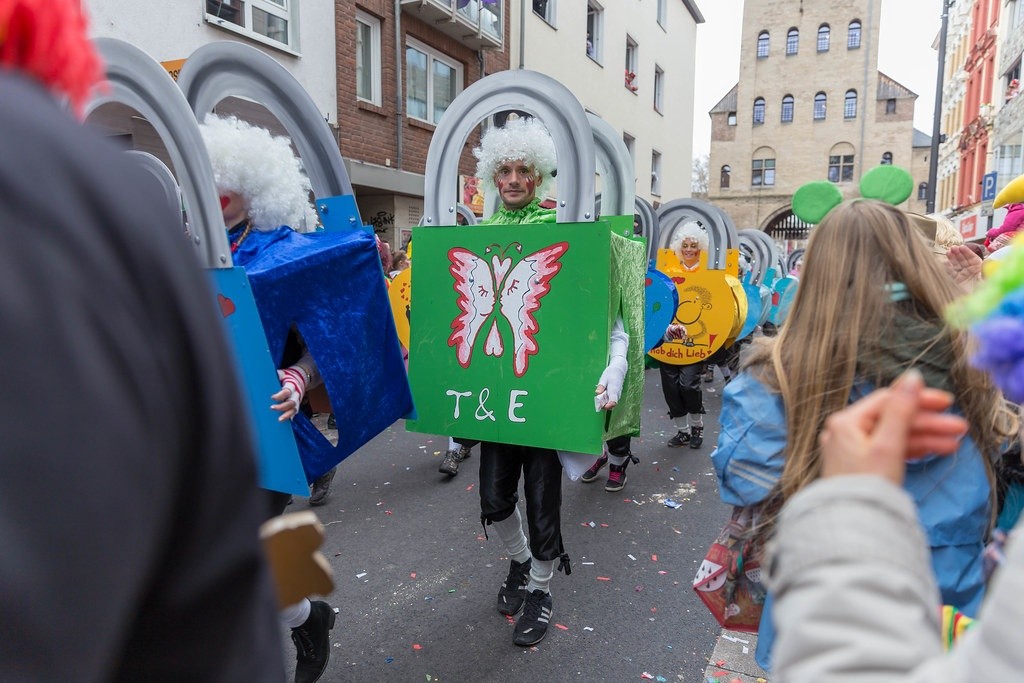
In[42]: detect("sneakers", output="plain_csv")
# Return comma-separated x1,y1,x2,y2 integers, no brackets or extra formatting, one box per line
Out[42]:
607,455,630,491
513,589,554,646
689,423,704,450
580,449,610,481
311,462,338,506
667,430,691,448
440,446,471,476
498,556,533,615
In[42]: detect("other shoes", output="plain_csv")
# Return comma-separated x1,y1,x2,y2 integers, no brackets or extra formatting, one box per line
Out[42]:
328,415,338,430
291,597,336,683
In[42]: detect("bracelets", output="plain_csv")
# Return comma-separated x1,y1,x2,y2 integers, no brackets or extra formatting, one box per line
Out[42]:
294,362,313,384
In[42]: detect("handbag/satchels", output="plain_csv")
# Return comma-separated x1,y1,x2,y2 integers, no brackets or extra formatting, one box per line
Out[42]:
692,494,780,632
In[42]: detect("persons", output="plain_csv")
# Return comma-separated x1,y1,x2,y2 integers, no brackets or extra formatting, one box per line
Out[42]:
656,218,803,451
704,194,1024,683
0,1,410,683
371,114,645,647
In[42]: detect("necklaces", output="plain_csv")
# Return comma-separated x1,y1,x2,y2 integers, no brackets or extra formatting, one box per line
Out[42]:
230,219,250,253
